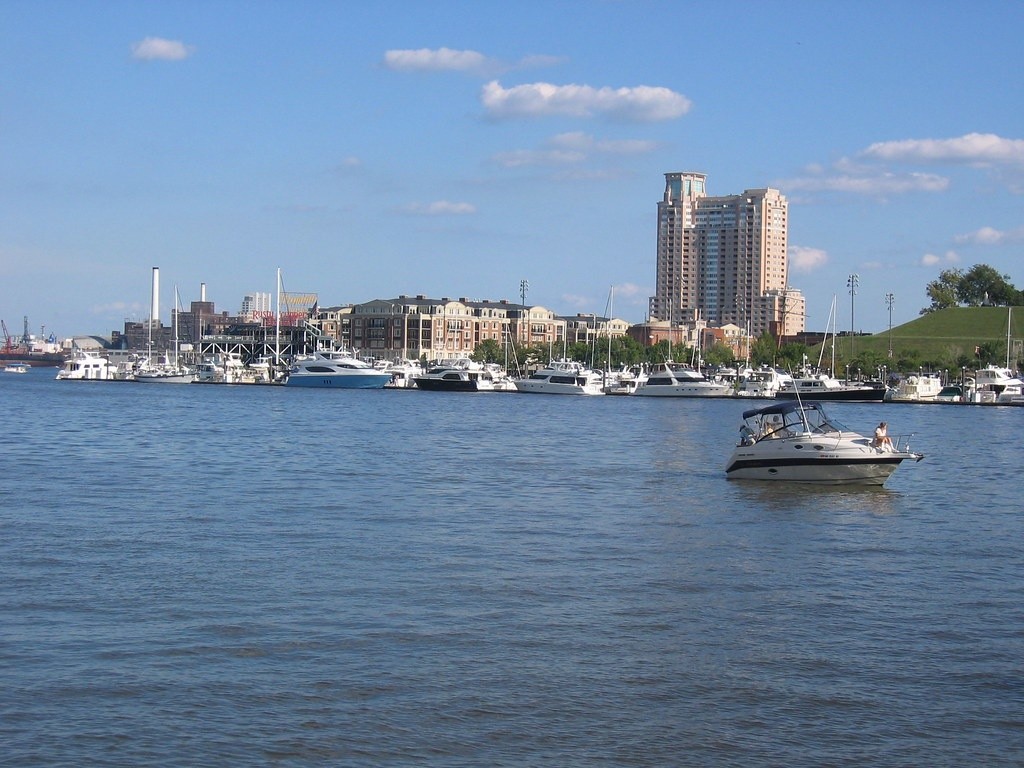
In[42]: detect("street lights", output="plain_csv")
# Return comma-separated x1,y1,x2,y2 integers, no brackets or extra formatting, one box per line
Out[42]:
847,273,860,362
884,293,895,360
518,279,529,350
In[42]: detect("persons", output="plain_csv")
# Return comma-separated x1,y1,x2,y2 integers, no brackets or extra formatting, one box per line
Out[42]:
874,422,900,452
739,416,782,446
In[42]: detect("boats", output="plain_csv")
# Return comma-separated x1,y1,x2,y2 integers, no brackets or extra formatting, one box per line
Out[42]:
5,361,32,373
55,265,1024,406
724,362,926,486
